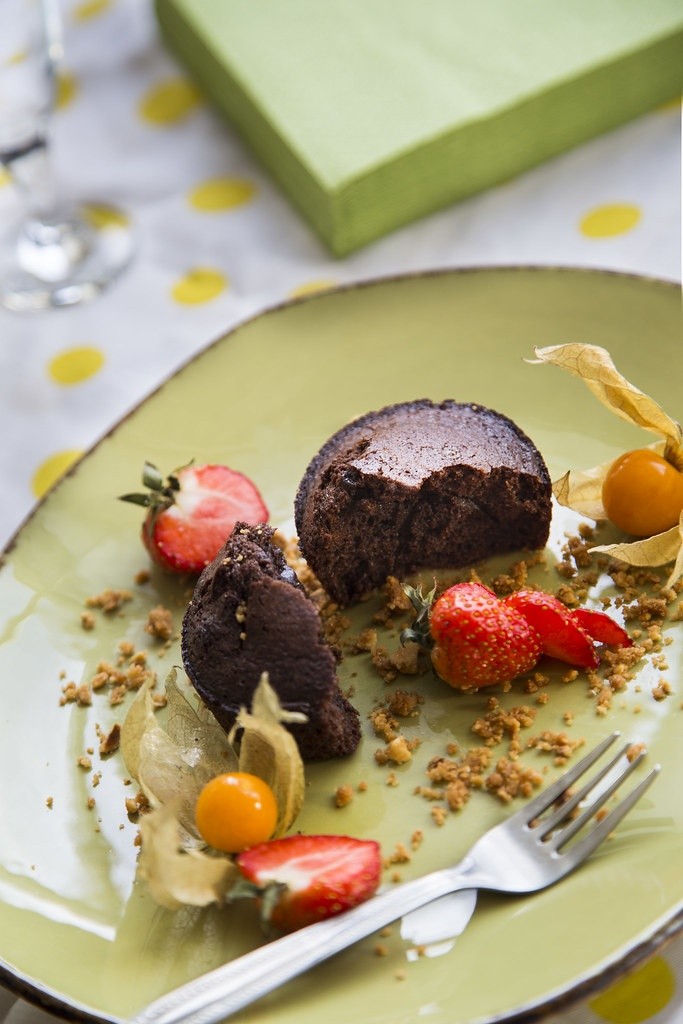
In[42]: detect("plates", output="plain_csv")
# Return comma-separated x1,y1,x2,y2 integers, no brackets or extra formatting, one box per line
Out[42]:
1,267,683,1024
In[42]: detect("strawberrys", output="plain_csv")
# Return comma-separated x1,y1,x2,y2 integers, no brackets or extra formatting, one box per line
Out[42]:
121,457,270,576
428,582,633,686
237,833,379,933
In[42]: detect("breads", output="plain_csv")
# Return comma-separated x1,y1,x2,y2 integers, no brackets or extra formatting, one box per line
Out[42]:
293,398,552,605
181,521,362,762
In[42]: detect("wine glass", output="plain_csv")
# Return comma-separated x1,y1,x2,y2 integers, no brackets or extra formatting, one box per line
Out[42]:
0,0,143,310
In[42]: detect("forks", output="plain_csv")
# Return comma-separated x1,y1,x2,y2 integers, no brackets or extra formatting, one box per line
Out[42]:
132,732,661,1024
